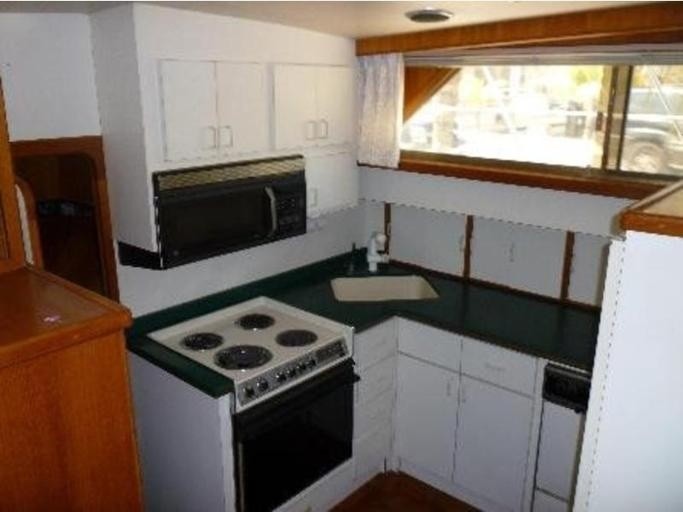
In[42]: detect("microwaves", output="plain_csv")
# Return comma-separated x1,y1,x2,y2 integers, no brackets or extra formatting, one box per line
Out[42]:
154,157,310,271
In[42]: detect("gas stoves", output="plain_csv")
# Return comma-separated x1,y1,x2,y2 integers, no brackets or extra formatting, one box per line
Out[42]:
145,294,356,416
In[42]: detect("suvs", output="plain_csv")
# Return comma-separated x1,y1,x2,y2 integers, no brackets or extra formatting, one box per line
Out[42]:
589,87,682,174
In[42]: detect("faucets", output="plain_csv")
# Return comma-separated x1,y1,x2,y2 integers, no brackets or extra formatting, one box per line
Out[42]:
366,230,391,274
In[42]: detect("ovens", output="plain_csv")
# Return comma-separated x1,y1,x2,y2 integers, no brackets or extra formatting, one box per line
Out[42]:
228,357,357,511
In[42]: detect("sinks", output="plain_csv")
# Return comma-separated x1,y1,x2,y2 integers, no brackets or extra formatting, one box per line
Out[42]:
330,273,440,303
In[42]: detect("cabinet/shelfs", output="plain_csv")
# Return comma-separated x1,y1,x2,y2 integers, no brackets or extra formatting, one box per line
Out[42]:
157,56,266,165
530,397,584,512
304,150,359,218
267,63,354,156
351,315,392,493
392,315,546,512
1,264,145,511
